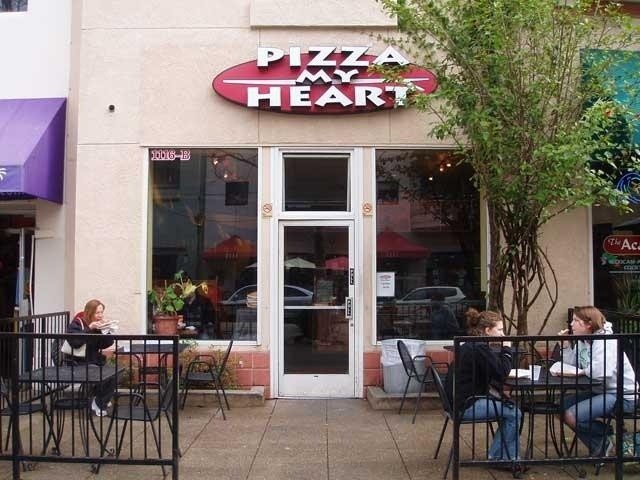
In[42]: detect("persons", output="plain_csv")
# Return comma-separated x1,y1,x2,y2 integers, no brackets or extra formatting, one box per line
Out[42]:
443,309,525,472
178,284,215,340
376,297,406,336
60,300,117,416
432,292,459,339
558,306,639,466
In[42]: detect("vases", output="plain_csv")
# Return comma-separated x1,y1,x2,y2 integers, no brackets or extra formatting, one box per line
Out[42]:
154,316,180,336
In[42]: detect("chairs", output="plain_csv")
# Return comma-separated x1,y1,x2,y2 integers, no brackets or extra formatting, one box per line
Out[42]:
0,334,234,480
397,339,640,480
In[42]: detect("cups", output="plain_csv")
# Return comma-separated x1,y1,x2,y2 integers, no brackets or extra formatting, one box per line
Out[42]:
124,342,131,352
527,364,542,380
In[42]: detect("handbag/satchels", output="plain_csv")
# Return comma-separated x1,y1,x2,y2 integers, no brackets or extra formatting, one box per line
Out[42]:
60,316,87,358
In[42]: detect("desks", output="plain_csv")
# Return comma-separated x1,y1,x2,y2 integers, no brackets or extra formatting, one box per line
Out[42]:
392,318,432,339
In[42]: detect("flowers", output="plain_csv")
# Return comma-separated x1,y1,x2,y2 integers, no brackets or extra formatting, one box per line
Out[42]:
147,270,209,314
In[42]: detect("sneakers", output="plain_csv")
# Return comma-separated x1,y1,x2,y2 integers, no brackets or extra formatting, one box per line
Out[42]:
498,465,531,472
594,441,614,466
93,396,112,417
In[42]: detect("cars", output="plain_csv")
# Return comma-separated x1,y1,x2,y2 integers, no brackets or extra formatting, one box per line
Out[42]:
214,281,315,323
202,307,305,347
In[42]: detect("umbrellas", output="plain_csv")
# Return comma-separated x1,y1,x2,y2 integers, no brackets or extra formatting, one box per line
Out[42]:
202,234,257,258
326,257,350,270
376,227,429,256
285,258,317,269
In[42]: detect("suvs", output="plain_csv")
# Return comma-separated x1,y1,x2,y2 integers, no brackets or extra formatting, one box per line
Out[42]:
377,284,468,320
